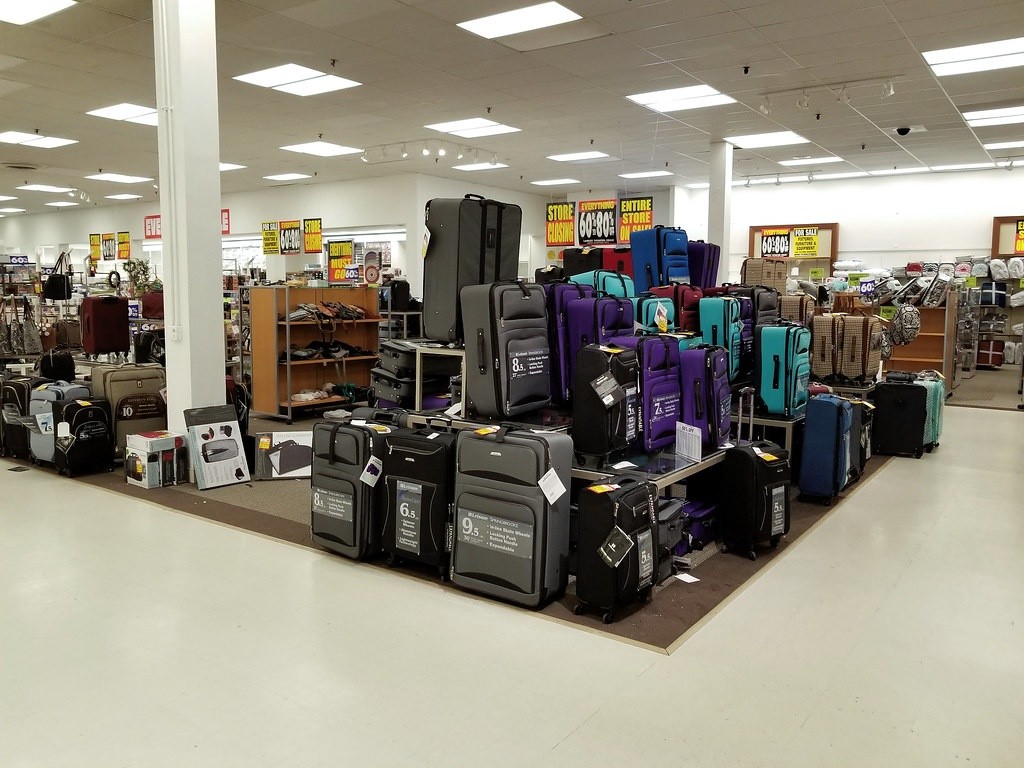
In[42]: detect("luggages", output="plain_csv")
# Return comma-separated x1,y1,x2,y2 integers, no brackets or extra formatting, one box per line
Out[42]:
91,363,166,451
29,380,91,466
79,294,130,359
52,398,114,478
756,318,811,420
310,194,945,625
0,375,54,458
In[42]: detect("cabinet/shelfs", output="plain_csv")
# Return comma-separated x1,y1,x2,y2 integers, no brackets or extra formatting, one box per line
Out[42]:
407,276,1024,574
0,263,387,425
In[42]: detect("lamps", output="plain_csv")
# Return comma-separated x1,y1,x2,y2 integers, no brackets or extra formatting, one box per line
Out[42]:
1004,158,1013,171
361,140,497,166
68,189,91,202
744,172,814,188
759,79,895,115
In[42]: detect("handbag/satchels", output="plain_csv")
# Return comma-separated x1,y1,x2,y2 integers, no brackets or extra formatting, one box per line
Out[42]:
0,294,81,384
276,300,375,408
132,284,165,364
42,252,72,300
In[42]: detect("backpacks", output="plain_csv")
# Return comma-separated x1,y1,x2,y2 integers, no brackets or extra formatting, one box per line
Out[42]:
881,291,921,365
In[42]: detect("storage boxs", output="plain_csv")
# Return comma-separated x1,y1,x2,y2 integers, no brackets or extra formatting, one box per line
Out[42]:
125,404,314,490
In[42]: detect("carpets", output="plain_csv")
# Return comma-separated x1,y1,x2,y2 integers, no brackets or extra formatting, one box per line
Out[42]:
945,363,1024,411
0,416,898,655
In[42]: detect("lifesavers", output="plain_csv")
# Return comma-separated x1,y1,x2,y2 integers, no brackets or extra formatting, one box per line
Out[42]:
108,271,120,288
